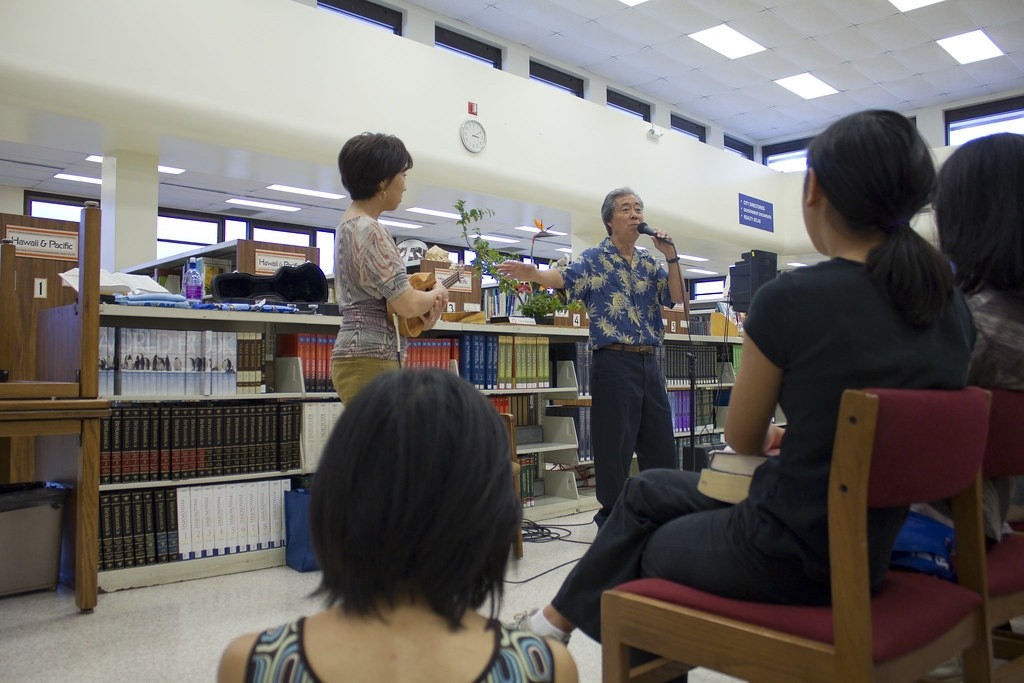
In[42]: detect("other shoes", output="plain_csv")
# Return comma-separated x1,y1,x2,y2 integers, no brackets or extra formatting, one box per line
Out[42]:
992,614,1024,662
502,607,571,646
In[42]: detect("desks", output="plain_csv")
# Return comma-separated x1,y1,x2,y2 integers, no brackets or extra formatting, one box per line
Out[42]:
0,409,111,614
0,201,109,410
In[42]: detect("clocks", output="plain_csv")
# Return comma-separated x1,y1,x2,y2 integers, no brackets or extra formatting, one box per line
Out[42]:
460,120,487,152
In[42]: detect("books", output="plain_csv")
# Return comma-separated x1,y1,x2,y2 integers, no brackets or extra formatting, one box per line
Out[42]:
97,326,742,573
183,257,232,297
483,288,528,318
690,316,711,336
59,269,170,296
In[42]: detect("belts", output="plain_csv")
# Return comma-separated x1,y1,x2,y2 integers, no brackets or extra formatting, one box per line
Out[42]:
605,342,657,355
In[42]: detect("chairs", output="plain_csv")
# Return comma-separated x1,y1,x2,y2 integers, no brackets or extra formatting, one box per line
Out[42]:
949,388,1024,683
601,387,993,683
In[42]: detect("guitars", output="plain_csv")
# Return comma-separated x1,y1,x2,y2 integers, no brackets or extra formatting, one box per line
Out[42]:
386,262,466,338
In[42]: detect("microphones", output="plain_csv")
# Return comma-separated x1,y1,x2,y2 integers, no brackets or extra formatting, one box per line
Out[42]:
637,222,674,246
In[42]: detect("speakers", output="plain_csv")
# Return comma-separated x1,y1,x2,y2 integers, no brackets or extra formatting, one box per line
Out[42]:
730,250,777,312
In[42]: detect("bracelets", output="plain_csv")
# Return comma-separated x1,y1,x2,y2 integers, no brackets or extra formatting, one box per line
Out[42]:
666,257,679,263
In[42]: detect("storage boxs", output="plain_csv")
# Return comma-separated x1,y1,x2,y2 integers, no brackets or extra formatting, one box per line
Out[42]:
212,263,340,316
516,426,543,445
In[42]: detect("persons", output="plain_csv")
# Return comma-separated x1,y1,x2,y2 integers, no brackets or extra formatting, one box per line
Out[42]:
935,133,1024,554
496,187,686,533
216,366,578,683
331,133,450,406
502,109,978,683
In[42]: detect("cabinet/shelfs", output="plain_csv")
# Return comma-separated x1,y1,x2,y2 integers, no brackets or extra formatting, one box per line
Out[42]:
95,238,744,593
